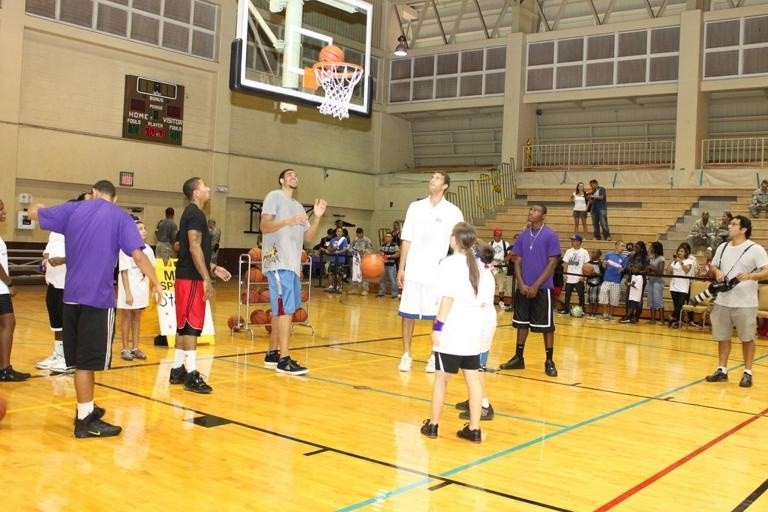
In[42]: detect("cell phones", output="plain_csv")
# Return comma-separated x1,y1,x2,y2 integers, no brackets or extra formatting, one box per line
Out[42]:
672,255,677,258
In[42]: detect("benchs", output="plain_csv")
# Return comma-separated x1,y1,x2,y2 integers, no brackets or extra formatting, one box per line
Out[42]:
473,186,768,310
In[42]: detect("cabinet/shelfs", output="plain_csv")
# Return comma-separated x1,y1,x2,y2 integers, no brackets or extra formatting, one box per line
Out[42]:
231,254,315,340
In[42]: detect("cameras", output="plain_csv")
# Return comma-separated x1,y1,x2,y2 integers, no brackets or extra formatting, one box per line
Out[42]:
709,275,739,294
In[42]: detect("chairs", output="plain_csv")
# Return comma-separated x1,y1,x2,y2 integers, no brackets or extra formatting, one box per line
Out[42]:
755,284,768,339
679,280,712,334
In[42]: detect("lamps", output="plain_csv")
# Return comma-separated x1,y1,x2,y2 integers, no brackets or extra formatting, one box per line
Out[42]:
394,35,408,57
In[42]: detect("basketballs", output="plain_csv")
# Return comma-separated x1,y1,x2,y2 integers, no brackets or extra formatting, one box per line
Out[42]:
583,263,594,276
363,252,385,277
320,45,344,70
229,248,308,333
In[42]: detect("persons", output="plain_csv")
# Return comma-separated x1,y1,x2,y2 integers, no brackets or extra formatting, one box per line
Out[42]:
260,170,328,374
0,201,31,382
555,179,767,387
26,177,231,438
318,219,404,300
398,170,562,442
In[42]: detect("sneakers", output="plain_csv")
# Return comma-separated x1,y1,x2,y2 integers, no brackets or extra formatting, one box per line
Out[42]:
121,349,135,362
35,354,75,375
131,347,148,361
420,418,438,438
0,364,32,382
500,356,525,369
457,426,482,443
184,370,214,395
399,353,412,373
499,301,508,310
545,361,557,377
348,288,401,299
455,399,495,421
706,368,728,382
592,237,611,240
323,286,342,294
170,365,190,385
276,355,309,376
559,308,701,330
740,372,753,388
73,405,127,439
425,355,436,374
263,349,281,369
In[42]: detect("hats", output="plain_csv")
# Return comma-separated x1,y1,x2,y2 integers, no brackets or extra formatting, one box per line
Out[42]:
494,230,502,236
569,235,583,241
